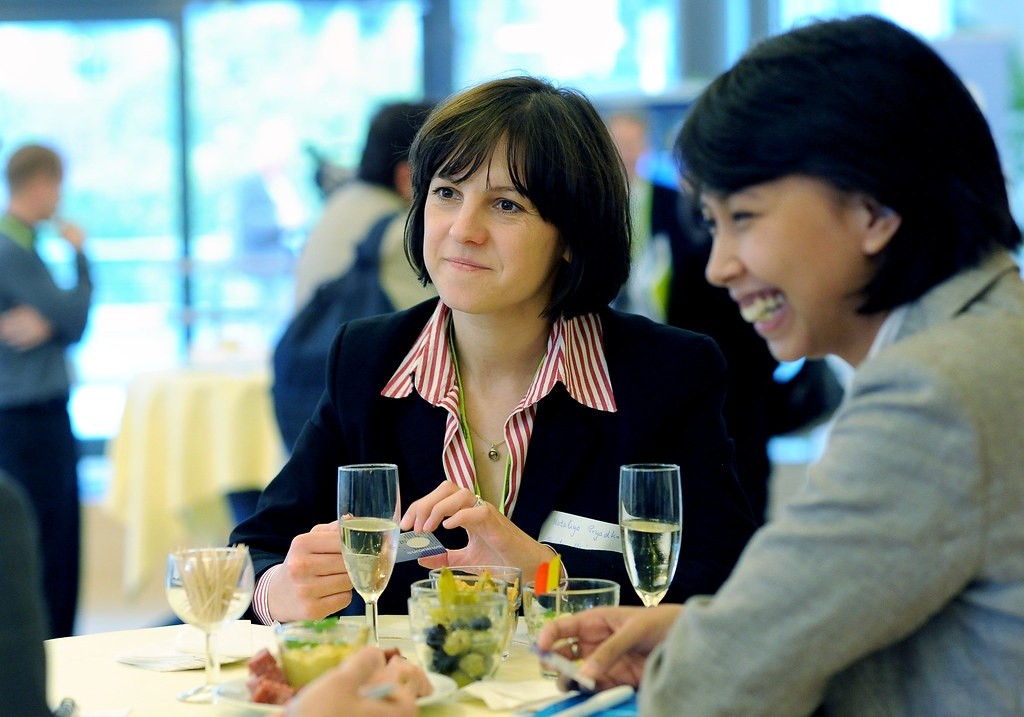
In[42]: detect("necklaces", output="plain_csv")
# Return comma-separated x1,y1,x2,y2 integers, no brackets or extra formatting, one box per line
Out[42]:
466,418,506,460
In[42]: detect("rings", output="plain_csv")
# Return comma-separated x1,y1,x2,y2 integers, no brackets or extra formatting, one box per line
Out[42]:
472,494,483,508
569,640,580,658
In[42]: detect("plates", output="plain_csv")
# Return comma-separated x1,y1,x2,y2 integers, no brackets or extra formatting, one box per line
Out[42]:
211,660,459,714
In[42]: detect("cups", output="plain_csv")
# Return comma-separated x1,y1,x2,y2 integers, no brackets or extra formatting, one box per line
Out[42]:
615,463,685,608
523,577,622,677
407,565,523,689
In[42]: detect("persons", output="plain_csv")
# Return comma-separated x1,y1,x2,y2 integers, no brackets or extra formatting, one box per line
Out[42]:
292,100,439,315
531,16,1022,716
0,144,95,639
603,113,698,329
224,68,769,625
268,644,420,716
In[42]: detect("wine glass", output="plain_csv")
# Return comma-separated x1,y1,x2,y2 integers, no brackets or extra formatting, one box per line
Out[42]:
166,546,256,703
333,459,403,651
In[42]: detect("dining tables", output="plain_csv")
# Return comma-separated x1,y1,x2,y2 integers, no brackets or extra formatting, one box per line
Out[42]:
42,612,645,717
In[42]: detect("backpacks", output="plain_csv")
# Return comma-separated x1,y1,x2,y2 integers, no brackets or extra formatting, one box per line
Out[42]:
270,210,401,464
